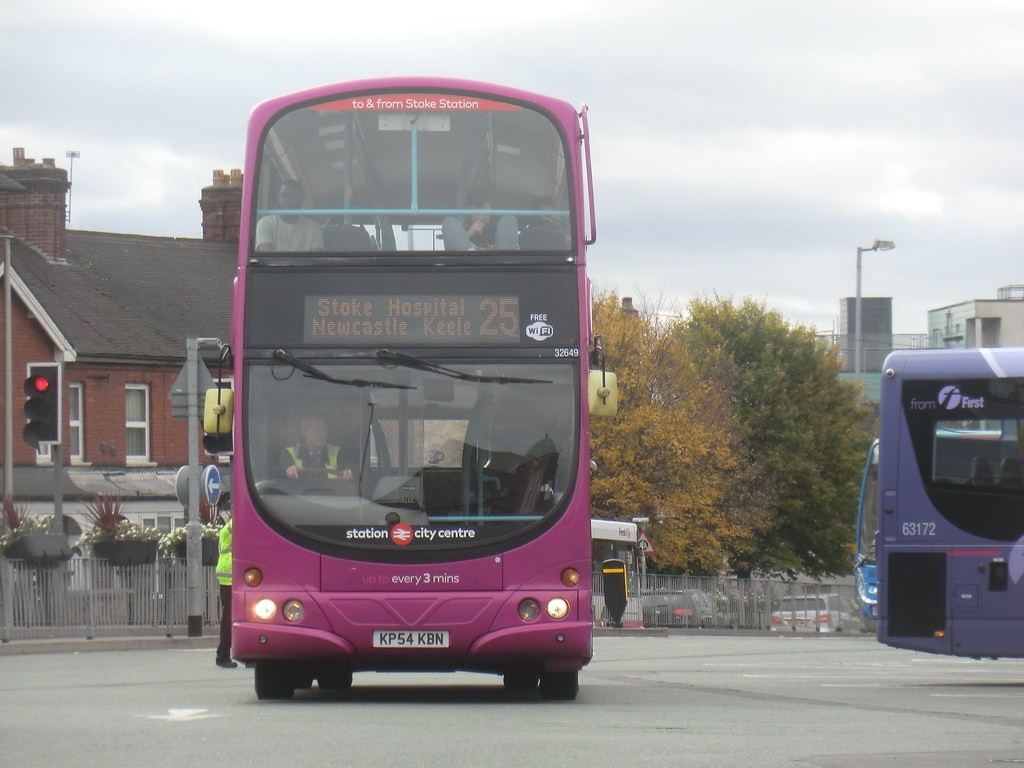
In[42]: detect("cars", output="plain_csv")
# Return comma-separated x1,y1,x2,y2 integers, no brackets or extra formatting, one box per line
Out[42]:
640,589,719,626
769,593,868,635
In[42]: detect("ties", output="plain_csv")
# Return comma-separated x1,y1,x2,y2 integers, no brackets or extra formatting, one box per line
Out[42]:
311,453,319,466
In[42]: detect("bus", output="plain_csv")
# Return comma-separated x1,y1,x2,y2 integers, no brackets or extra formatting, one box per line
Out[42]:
202,75,623,700
589,519,645,628
869,344,1024,664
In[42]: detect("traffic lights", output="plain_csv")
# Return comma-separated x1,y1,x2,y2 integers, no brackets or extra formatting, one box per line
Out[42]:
21,361,62,445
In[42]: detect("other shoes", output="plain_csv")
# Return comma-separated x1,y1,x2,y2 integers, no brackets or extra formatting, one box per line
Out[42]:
216,658,238,668
247,663,255,668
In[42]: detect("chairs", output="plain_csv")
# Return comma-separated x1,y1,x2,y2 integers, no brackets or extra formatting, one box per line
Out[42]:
442,216,477,250
998,457,1024,490
969,456,995,492
321,223,377,251
492,215,520,250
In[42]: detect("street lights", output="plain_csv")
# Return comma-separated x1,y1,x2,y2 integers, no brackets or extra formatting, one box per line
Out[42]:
853,240,895,403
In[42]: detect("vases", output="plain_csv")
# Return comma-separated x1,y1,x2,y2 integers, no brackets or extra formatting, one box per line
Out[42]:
93,540,157,566
177,540,220,566
4,533,72,570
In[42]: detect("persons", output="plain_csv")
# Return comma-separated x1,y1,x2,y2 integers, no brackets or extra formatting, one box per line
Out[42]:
272,409,355,485
529,198,571,241
215,519,239,668
441,186,519,251
254,179,325,252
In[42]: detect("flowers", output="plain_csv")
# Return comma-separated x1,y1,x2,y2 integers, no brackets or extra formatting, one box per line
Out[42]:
75,518,164,547
159,522,224,561
0,513,55,551
